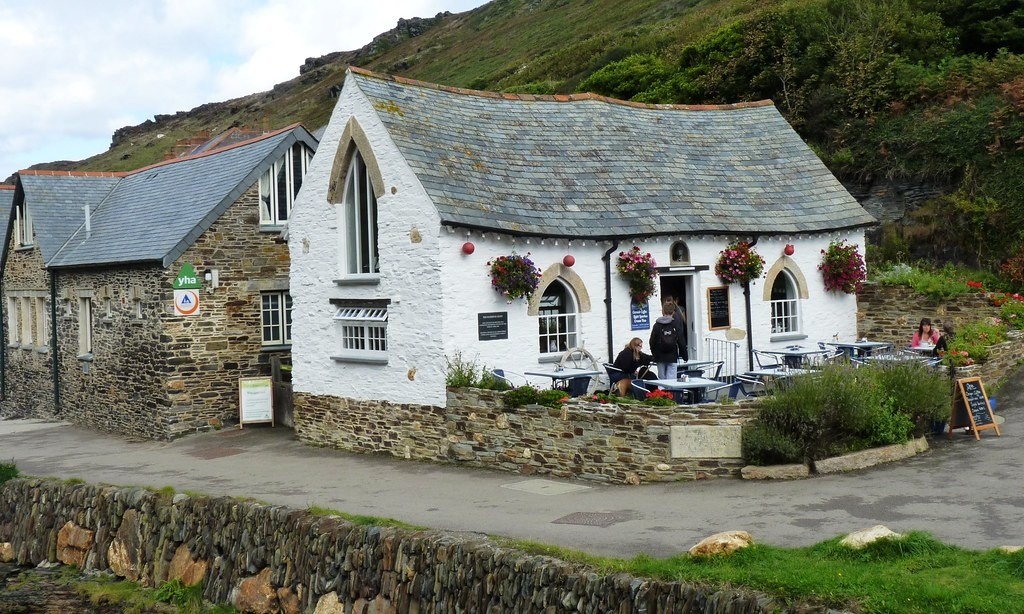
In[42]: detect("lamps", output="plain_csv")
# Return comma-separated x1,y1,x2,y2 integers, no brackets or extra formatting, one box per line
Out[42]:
204,267,214,293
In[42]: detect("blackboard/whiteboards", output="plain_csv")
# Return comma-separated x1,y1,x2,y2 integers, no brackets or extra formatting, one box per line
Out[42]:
959,376,997,429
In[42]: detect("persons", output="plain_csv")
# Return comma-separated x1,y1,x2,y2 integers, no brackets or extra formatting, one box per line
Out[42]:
609,337,654,382
932,321,958,380
911,318,939,369
660,295,685,357
649,303,688,380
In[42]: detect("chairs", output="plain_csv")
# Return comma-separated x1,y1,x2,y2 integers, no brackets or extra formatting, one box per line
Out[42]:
555,376,592,398
603,360,775,404
493,368,530,391
753,342,943,383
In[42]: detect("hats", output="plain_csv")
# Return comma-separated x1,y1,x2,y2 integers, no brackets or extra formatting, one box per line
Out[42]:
921,318,933,325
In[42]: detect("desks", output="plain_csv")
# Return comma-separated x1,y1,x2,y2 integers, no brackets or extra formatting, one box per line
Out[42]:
650,359,715,368
863,355,930,363
828,341,889,357
905,344,936,352
745,367,823,388
761,348,831,368
523,369,604,390
642,377,727,403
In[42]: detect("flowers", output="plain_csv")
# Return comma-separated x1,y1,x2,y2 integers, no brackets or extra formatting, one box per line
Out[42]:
816,234,870,296
616,244,661,309
714,237,769,287
484,248,544,302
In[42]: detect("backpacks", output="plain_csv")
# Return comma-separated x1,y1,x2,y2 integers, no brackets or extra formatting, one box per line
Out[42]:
658,324,677,353
